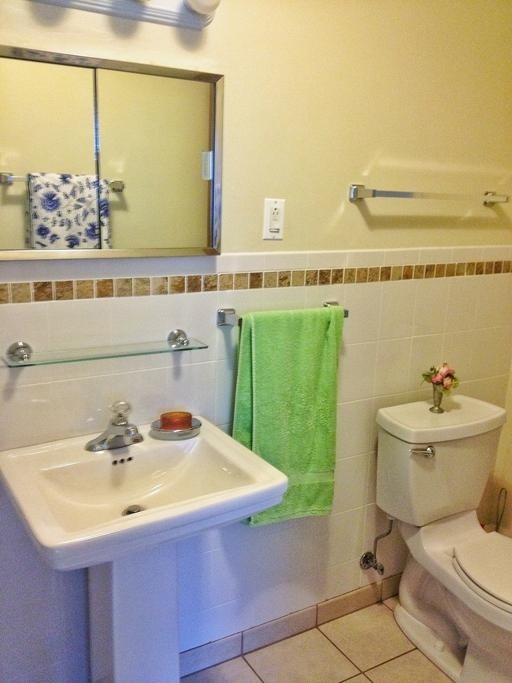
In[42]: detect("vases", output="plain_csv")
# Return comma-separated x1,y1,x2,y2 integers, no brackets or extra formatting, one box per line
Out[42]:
431,384,448,415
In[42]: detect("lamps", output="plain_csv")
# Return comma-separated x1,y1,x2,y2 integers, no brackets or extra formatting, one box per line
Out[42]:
185,0,221,21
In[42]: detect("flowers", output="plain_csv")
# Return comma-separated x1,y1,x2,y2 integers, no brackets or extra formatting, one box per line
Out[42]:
419,363,460,397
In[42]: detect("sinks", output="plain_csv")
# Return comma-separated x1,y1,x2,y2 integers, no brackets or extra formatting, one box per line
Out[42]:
0,416,289,574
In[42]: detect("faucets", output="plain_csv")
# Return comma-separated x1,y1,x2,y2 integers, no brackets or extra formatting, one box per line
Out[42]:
84,399,142,451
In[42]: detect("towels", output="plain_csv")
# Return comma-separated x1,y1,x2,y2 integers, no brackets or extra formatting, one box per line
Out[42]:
229,301,346,532
22,169,115,252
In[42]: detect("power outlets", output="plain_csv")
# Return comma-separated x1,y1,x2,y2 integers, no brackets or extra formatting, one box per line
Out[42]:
260,196,286,243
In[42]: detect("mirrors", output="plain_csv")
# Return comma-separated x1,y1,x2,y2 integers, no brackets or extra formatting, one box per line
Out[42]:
0,43,228,267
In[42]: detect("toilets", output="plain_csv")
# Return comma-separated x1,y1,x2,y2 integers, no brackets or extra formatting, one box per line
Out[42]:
376,397,512,683
